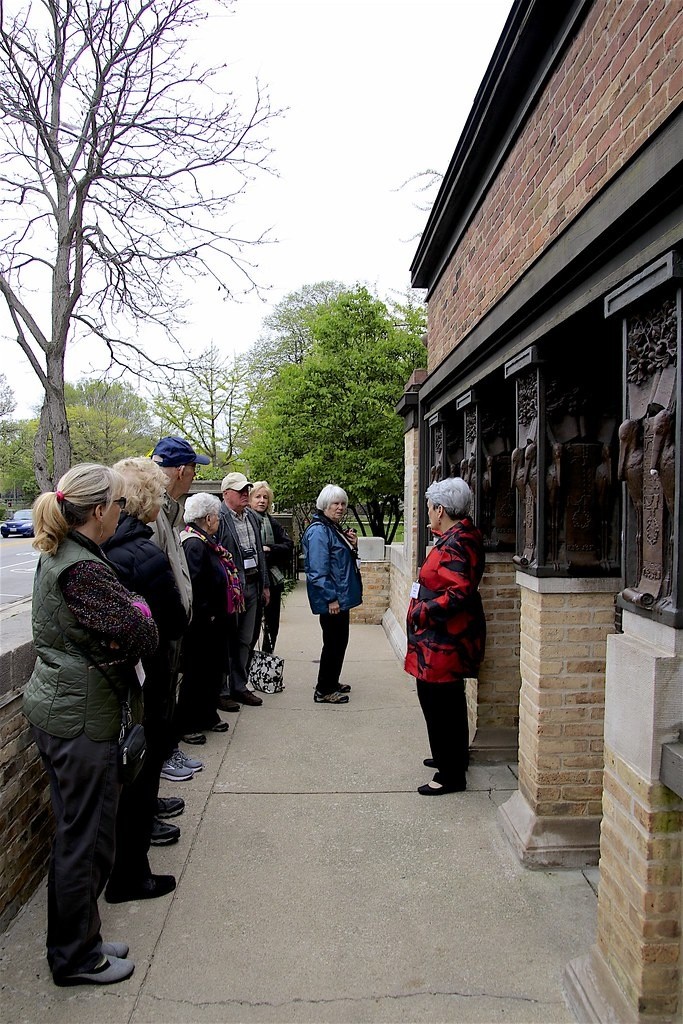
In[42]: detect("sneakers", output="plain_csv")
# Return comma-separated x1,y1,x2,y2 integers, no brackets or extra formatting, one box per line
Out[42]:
156,797,186,818
159,760,195,781
314,690,350,703
182,732,207,744
150,817,180,846
172,750,205,772
336,683,351,692
209,721,229,731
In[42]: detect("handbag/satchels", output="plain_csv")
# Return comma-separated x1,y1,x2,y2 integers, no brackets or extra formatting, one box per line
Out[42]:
249,650,285,694
117,725,147,781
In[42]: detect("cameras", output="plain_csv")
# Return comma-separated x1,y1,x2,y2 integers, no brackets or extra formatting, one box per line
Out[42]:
241,547,260,575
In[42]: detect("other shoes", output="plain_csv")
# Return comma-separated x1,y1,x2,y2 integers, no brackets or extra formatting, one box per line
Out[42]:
423,758,437,768
51,954,135,987
99,939,129,958
228,691,263,706
217,696,241,712
418,784,466,795
104,874,176,904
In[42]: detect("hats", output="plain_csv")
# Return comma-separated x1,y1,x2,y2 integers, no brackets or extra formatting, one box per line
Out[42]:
151,435,211,467
221,472,254,491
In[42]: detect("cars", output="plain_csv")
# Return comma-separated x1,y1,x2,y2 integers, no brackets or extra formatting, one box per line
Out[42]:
0,508,35,538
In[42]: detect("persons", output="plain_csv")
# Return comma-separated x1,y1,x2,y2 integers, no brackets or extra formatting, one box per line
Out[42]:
301,484,363,703
24,462,160,987
99,436,294,903
405,477,486,796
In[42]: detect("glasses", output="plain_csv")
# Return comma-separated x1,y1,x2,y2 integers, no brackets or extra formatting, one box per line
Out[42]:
92,496,127,517
